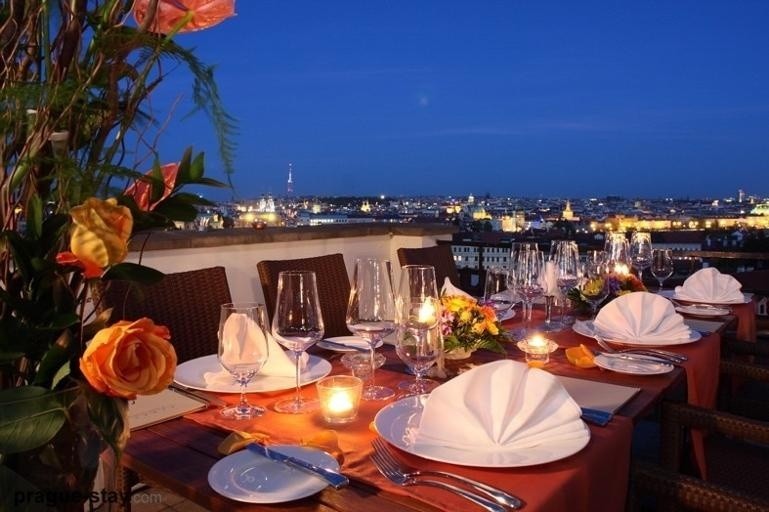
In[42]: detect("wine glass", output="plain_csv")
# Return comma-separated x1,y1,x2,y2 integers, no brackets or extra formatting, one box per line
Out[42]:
352,259,397,401
402,262,442,397
217,303,264,418
487,237,682,358
278,272,325,415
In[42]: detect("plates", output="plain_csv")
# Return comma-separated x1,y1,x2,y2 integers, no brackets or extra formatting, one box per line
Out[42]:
570,307,726,375
315,334,387,354
207,446,344,502
176,353,330,395
379,392,592,468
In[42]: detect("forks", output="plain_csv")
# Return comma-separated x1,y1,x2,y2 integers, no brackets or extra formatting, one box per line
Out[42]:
365,437,523,511
588,330,679,369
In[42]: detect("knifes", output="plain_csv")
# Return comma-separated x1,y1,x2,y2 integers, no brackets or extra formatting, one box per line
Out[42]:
245,443,349,488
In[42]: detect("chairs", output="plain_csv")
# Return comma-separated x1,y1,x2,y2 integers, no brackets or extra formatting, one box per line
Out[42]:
397,244,459,298
93,266,237,365
257,254,351,338
625,318,769,512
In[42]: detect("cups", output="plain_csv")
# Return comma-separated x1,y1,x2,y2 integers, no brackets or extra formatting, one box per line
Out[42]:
321,376,361,423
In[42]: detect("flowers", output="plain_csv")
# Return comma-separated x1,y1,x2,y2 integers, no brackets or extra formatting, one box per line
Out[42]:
1,0,238,512
